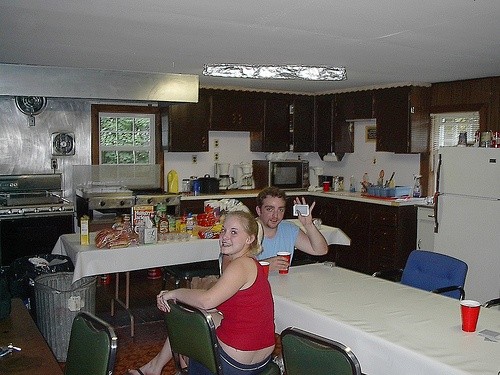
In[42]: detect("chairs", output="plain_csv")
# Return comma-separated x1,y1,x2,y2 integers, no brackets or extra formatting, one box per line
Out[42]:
62,310,118,375
166,300,281,375
281,328,361,375
372,250,468,299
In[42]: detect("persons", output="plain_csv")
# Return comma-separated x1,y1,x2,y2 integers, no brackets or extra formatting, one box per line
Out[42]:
255,187,328,271
128,211,276,375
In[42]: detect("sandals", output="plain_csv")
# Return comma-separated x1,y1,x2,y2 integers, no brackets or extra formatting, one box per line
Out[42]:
123,368,144,375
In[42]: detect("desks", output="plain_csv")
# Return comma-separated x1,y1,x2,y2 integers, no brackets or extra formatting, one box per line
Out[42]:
51,218,350,336
0,299,64,375
267,262,500,375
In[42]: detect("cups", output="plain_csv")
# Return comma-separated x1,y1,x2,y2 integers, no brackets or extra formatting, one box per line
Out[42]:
259,262,270,279
459,299,481,332
323,182,330,192
277,251,291,274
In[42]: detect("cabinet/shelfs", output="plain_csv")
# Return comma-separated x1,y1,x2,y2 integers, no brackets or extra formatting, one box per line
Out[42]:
180,195,434,283
163,76,500,160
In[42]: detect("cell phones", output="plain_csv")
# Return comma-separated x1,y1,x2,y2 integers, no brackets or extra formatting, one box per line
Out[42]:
293,204,309,216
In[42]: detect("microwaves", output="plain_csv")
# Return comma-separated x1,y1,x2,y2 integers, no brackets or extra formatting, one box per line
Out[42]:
251,159,309,191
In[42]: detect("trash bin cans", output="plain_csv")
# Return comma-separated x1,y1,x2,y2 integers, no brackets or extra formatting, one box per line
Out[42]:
34,272,96,363
22,254,74,314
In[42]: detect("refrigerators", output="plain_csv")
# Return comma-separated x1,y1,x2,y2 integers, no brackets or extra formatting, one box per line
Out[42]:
433,147,500,310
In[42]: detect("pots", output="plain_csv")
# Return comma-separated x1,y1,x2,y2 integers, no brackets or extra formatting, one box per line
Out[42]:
197,174,221,194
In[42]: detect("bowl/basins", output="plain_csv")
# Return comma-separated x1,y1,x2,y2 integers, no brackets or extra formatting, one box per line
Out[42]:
318,175,334,188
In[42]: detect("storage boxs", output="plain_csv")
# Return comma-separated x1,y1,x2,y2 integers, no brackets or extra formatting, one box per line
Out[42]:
368,185,410,197
130,205,154,234
80,217,89,245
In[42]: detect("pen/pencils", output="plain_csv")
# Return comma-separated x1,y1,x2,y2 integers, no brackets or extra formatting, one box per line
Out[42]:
388,172,395,183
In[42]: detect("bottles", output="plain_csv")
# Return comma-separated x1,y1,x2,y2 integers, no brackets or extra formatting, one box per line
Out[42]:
167,168,178,193
363,173,369,183
349,175,356,192
182,176,200,197
158,211,198,235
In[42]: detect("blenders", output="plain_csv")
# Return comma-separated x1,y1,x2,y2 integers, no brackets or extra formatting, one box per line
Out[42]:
237,161,255,189
214,163,234,190
